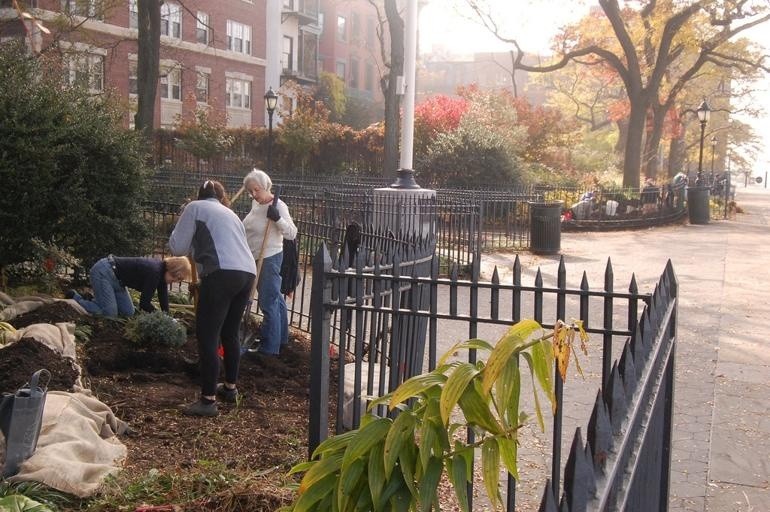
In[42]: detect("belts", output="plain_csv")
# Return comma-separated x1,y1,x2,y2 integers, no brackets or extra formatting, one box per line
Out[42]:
107,254,125,289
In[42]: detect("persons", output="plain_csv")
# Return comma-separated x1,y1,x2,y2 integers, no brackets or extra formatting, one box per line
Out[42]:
242,170,298,356
568,182,674,220
65,255,192,320
169,181,258,416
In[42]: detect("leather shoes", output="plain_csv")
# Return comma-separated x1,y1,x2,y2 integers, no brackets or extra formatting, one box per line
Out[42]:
247,348,272,361
252,338,288,354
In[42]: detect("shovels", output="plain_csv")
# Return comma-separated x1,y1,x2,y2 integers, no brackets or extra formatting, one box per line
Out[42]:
238,184,283,356
189,254,220,388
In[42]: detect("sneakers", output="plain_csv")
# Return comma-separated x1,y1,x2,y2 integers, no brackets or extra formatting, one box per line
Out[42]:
64,289,94,301
181,396,219,417
215,381,238,403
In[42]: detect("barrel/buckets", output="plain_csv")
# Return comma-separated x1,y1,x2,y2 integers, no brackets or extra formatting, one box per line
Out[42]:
531,199,564,253
342,363,390,431
606,200,619,216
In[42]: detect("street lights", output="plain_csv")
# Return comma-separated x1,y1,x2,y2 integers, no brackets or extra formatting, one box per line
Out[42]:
708,134,718,185
693,96,712,187
262,85,279,178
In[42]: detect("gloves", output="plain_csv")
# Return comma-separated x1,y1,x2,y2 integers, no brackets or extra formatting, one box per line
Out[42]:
267,205,281,223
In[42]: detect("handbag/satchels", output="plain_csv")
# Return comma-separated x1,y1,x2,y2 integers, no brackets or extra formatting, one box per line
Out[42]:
0,368,51,479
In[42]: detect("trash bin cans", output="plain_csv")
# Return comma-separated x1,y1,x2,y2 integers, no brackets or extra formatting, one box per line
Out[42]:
527,199,564,255
